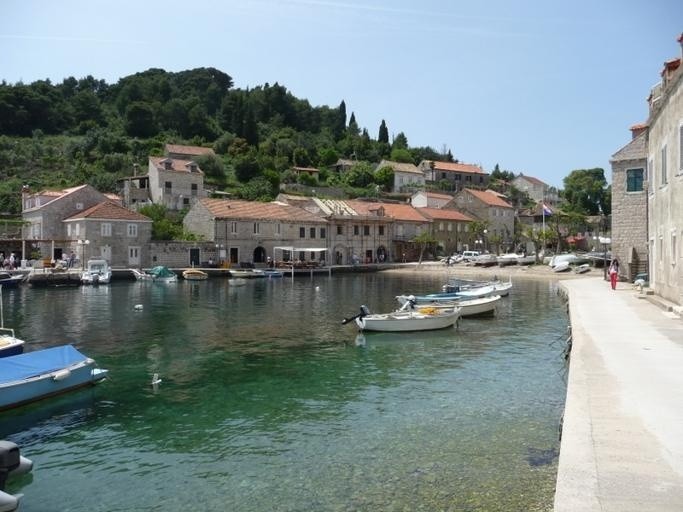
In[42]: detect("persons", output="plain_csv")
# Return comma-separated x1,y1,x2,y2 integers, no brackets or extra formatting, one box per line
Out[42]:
608,259,620,290
69,250,76,268
0,251,17,270
266,255,271,268
62,252,68,266
353,253,360,265
208,257,213,264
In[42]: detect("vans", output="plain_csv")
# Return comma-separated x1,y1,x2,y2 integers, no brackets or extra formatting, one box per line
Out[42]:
463,250,479,262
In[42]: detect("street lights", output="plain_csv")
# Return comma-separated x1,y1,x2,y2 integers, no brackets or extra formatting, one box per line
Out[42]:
78,240,90,270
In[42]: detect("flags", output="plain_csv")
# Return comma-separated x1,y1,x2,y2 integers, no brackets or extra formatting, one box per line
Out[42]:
543,204,553,216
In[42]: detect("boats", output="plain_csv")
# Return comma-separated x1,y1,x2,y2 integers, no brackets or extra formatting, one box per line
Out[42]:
183,270,209,282
130,266,178,282
0,326,108,413
231,269,282,279
0,272,23,288
343,280,512,332
480,253,537,266
83,259,111,283
